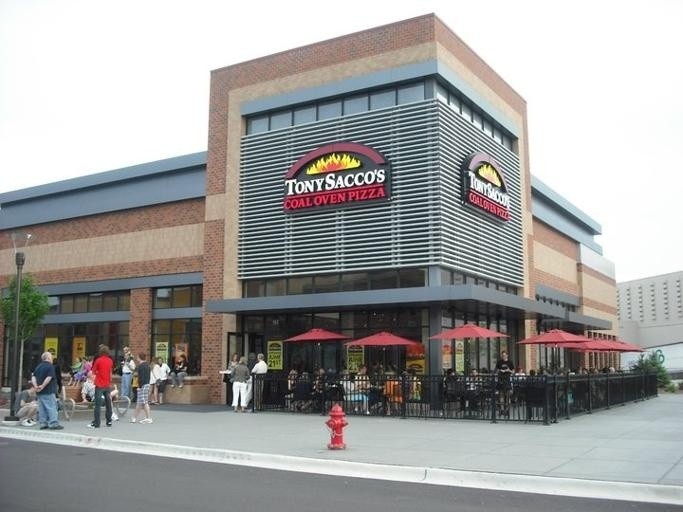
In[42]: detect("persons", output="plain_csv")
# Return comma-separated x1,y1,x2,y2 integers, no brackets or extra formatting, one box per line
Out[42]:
13,344,268,430
283,351,616,415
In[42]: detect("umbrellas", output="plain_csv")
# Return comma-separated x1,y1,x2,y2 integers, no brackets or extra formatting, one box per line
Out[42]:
282,327,353,373
515,329,646,368
343,331,419,372
427,322,511,376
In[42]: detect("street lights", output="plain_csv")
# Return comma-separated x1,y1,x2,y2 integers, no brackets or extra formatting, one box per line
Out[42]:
3,232,38,423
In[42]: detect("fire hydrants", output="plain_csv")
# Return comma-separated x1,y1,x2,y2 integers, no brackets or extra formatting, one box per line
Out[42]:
324,403,349,451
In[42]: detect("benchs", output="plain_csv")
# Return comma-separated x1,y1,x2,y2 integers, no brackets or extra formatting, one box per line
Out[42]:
58,384,132,422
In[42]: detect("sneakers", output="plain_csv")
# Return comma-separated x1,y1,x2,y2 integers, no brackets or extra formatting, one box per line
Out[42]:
111,412,120,421
128,416,137,423
19,418,37,427
85,421,112,428
138,417,152,424
40,425,63,430
